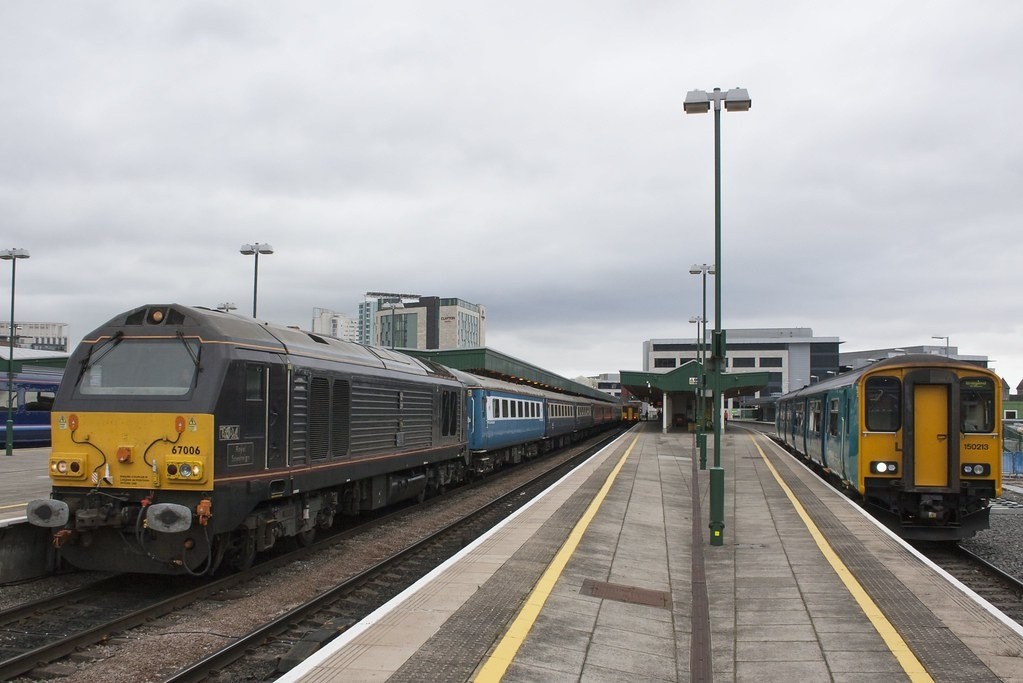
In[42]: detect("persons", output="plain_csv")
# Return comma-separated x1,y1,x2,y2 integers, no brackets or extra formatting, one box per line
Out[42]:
724,410,729,425
657,410,662,422
644,410,649,422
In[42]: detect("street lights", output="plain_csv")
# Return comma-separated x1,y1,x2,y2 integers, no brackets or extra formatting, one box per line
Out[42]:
239,242,274,321
689,315,709,449
795,348,908,388
932,336,949,358
688,263,716,470
682,85,755,546
216,303,240,314
0,247,31,457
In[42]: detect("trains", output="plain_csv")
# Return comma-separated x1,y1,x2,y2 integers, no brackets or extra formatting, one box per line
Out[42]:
22,302,623,583
0,371,70,451
772,353,1005,546
620,403,642,424
